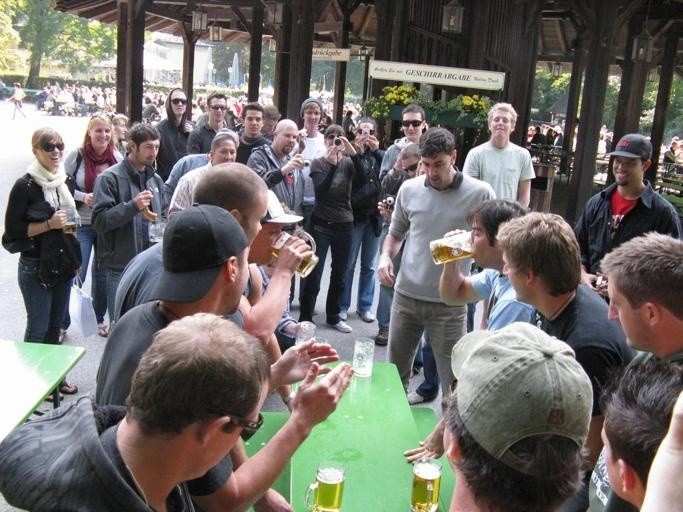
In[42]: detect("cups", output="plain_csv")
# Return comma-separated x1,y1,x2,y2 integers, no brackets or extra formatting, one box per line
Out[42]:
147,219,166,242
294,320,317,347
57,206,77,233
429,229,476,265
408,457,443,511
304,460,345,511
270,229,319,279
352,337,375,378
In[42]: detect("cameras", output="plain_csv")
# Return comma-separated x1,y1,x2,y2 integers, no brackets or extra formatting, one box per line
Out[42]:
363,129,370,141
302,158,313,168
334,138,343,150
385,195,395,211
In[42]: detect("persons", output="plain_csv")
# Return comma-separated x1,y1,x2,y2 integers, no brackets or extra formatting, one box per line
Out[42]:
298,125,367,334
603,364,683,511
240,191,320,352
589,234,683,510
245,119,311,248
338,118,384,321
187,94,230,156
408,156,476,408
114,163,269,342
1,313,269,512
404,200,535,465
442,323,593,512
235,104,274,166
9,83,26,120
152,88,194,184
56,116,124,344
376,128,497,425
376,105,425,345
542,122,567,146
288,98,326,312
261,105,281,144
168,134,236,231
496,214,633,511
531,127,545,145
108,114,128,159
163,129,240,207
571,134,682,312
663,141,678,173
94,203,354,512
2,129,80,345
34,81,165,118
591,123,616,175
378,143,421,220
462,103,535,206
322,95,364,144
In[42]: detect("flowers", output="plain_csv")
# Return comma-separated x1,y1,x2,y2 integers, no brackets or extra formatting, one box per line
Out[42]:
445,94,494,144
364,83,430,121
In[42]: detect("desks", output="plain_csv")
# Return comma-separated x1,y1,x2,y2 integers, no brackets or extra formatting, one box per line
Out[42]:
240,360,455,511
0,340,86,444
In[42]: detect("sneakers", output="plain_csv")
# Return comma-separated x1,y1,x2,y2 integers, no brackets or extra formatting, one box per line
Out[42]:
338,311,348,319
408,392,426,403
375,327,390,345
359,311,376,322
327,321,352,332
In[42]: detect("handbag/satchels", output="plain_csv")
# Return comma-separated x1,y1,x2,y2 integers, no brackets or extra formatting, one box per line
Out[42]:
304,207,315,237
40,231,83,287
68,273,98,338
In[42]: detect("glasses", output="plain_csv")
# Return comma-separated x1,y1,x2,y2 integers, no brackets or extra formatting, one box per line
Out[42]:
229,413,262,439
38,143,64,152
171,99,188,105
358,129,377,134
403,119,425,127
328,135,342,139
209,104,228,110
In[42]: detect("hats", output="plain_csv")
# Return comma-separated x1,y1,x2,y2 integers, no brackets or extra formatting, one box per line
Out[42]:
260,189,304,223
452,322,594,474
146,203,248,302
609,133,653,159
300,98,324,121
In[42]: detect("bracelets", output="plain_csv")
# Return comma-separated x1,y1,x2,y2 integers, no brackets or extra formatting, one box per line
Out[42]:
45,219,51,230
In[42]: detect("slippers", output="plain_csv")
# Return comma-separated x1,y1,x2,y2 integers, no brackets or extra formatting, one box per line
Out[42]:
59,383,78,394
98,325,109,337
45,391,64,402
